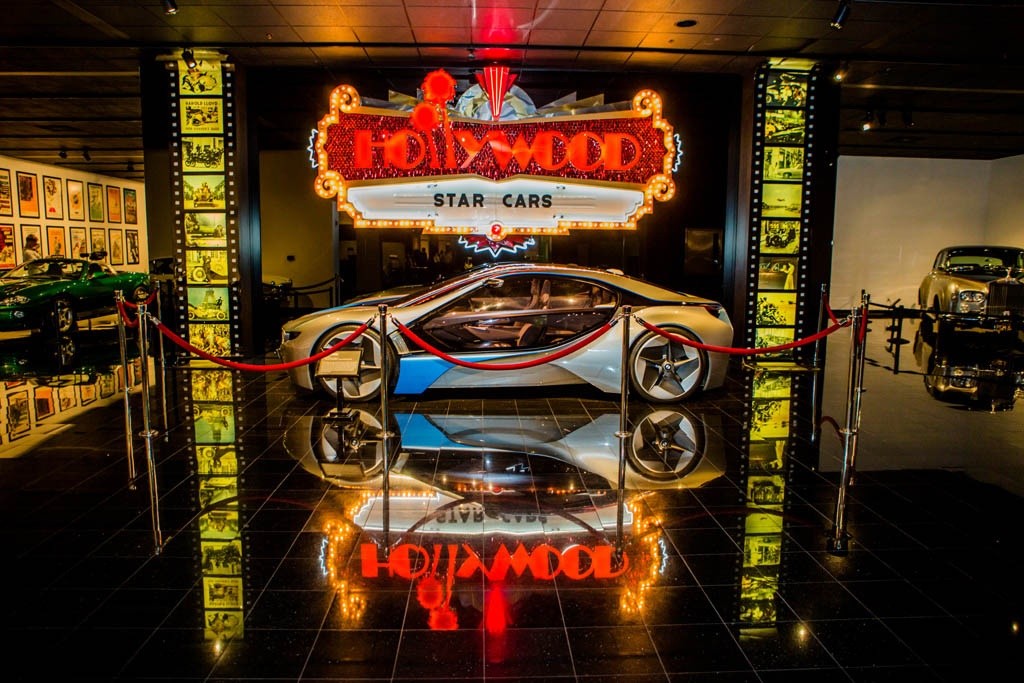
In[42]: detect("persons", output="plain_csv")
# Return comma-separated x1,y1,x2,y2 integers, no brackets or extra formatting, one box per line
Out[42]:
23,234,45,274
336,243,474,299
177,70,226,425
45,243,65,273
749,78,807,396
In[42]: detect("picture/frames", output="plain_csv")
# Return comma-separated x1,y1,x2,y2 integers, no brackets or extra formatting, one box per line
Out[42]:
4,357,144,442
0,167,145,271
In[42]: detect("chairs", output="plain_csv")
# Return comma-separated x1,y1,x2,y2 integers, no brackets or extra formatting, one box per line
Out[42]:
465,279,552,348
557,286,613,334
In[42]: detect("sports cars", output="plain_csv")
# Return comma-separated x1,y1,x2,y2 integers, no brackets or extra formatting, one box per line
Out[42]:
918,246,1024,340
281,260,735,403
0,256,147,332
912,325,1024,411
284,400,723,513
